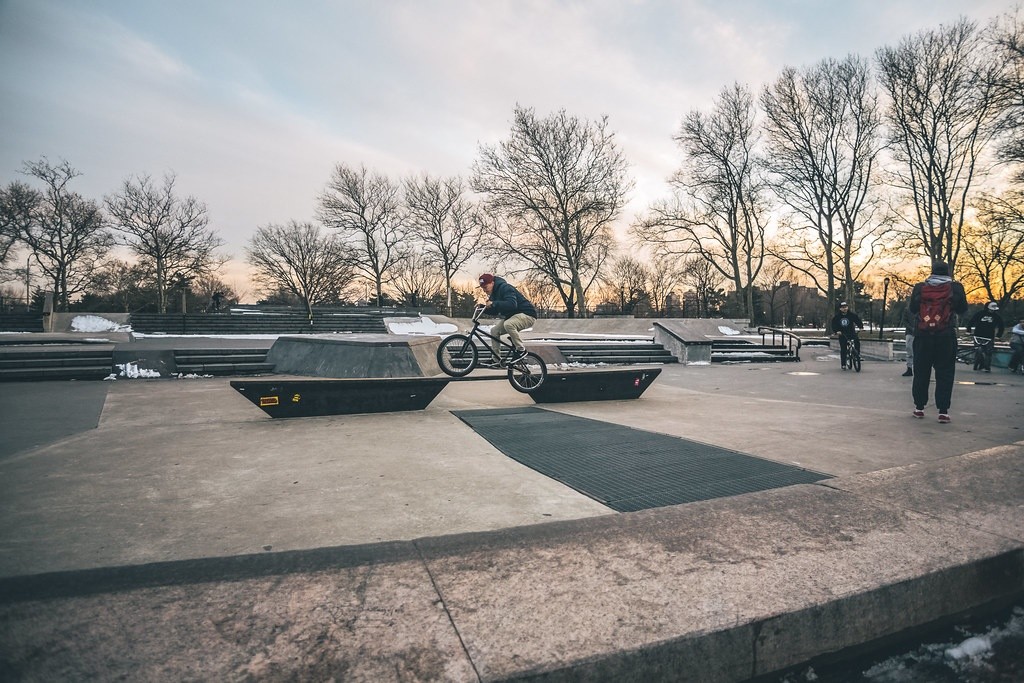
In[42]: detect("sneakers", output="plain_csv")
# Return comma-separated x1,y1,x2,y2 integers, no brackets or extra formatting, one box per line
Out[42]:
510,349,528,364
480,358,498,368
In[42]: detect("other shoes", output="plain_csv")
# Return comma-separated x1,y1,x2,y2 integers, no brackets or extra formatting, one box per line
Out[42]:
937,413,951,423
840,361,846,371
911,406,925,418
902,367,913,376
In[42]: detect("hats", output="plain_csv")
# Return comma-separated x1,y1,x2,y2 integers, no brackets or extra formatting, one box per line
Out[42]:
987,302,999,310
838,300,848,307
479,274,494,288
932,258,949,275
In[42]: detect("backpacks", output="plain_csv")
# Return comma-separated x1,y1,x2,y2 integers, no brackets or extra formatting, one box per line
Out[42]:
918,282,955,331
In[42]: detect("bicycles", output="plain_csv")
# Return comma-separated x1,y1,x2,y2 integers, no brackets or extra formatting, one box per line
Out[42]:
1008,331,1024,375
436,306,547,393
965,332,1000,370
835,329,866,372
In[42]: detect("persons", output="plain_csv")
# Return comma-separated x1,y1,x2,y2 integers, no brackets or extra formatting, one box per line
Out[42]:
474,273,537,367
412,290,418,306
902,297,916,377
831,302,864,372
1008,319,1024,372
212,292,224,309
909,262,969,423
966,301,1004,372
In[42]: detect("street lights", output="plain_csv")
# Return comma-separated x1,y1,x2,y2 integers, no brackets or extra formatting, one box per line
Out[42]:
621,286,624,315
27,253,40,312
879,278,890,339
1014,296,1016,323
683,297,685,318
870,298,873,334
